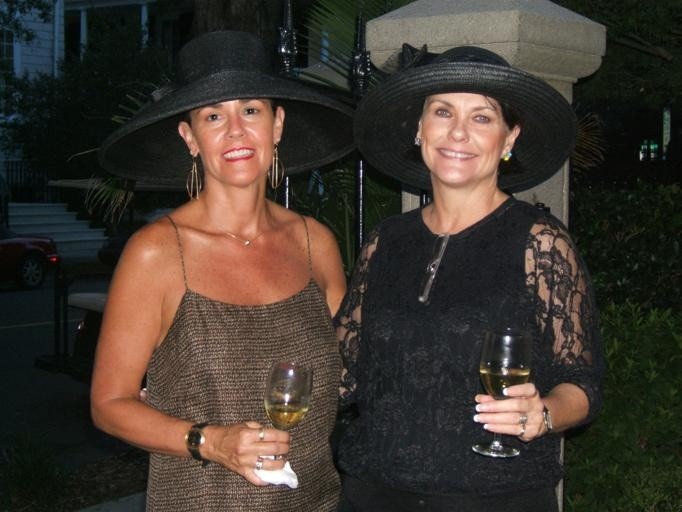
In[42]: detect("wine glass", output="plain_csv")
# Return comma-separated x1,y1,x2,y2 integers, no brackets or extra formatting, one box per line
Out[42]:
469,328,531,459
263,360,314,462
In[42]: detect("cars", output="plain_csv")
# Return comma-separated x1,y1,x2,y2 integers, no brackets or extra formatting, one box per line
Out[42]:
0,225,60,292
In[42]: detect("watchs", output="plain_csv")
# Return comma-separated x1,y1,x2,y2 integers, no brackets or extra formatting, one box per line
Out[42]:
186,420,210,467
543,404,553,434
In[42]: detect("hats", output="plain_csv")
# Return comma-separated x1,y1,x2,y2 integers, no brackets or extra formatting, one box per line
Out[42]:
97,29,360,191
353,46,576,193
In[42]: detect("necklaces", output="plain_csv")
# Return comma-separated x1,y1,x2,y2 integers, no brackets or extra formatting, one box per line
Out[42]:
216,225,264,246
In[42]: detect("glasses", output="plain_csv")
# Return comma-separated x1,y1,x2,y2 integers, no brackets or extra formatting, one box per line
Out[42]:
419,233,450,307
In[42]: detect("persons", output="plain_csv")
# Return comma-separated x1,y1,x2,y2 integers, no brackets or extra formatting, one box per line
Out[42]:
328,45,605,512
90,31,347,511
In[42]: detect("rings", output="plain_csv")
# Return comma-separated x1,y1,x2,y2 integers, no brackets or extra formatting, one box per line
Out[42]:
520,413,528,425
256,456,264,470
258,426,264,441
521,424,526,436
